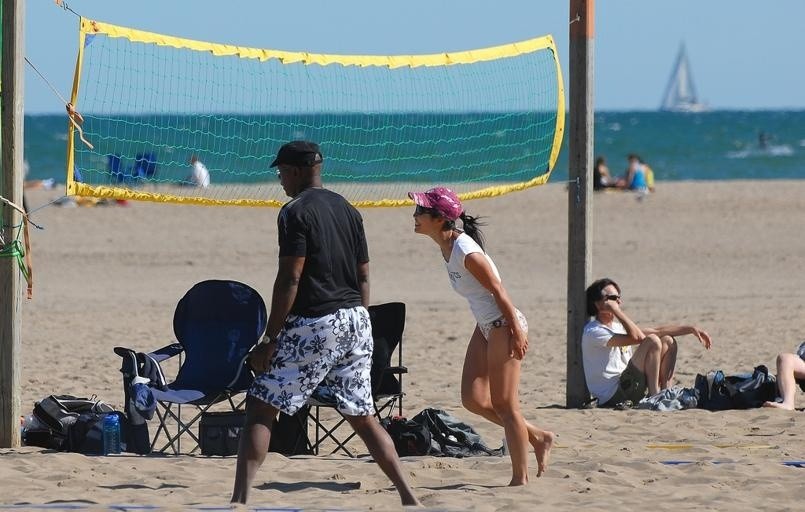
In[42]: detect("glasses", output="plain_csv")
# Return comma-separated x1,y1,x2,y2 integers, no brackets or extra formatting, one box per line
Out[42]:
415,205,432,216
274,168,291,176
604,294,621,301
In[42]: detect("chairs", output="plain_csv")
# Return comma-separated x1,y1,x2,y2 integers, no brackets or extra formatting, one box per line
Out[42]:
137,278,268,458
300,301,409,456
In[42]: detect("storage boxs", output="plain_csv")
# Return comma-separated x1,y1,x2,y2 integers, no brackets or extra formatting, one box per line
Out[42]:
198,412,278,455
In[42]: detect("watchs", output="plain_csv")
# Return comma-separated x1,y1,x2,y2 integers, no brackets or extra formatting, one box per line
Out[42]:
258,330,276,343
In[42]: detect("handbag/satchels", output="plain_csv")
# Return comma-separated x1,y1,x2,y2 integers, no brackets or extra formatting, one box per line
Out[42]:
21,394,123,452
693,370,774,411
384,404,485,457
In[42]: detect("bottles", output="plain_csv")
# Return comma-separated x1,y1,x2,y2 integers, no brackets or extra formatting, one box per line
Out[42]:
101,414,121,456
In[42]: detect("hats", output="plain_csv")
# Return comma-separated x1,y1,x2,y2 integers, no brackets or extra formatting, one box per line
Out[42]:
408,186,464,222
268,141,324,169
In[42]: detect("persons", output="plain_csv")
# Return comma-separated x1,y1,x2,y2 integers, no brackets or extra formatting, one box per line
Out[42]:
229,141,423,510
181,155,212,187
624,153,646,191
582,278,712,404
638,158,655,184
402,185,554,487
764,344,805,410
593,155,613,190
